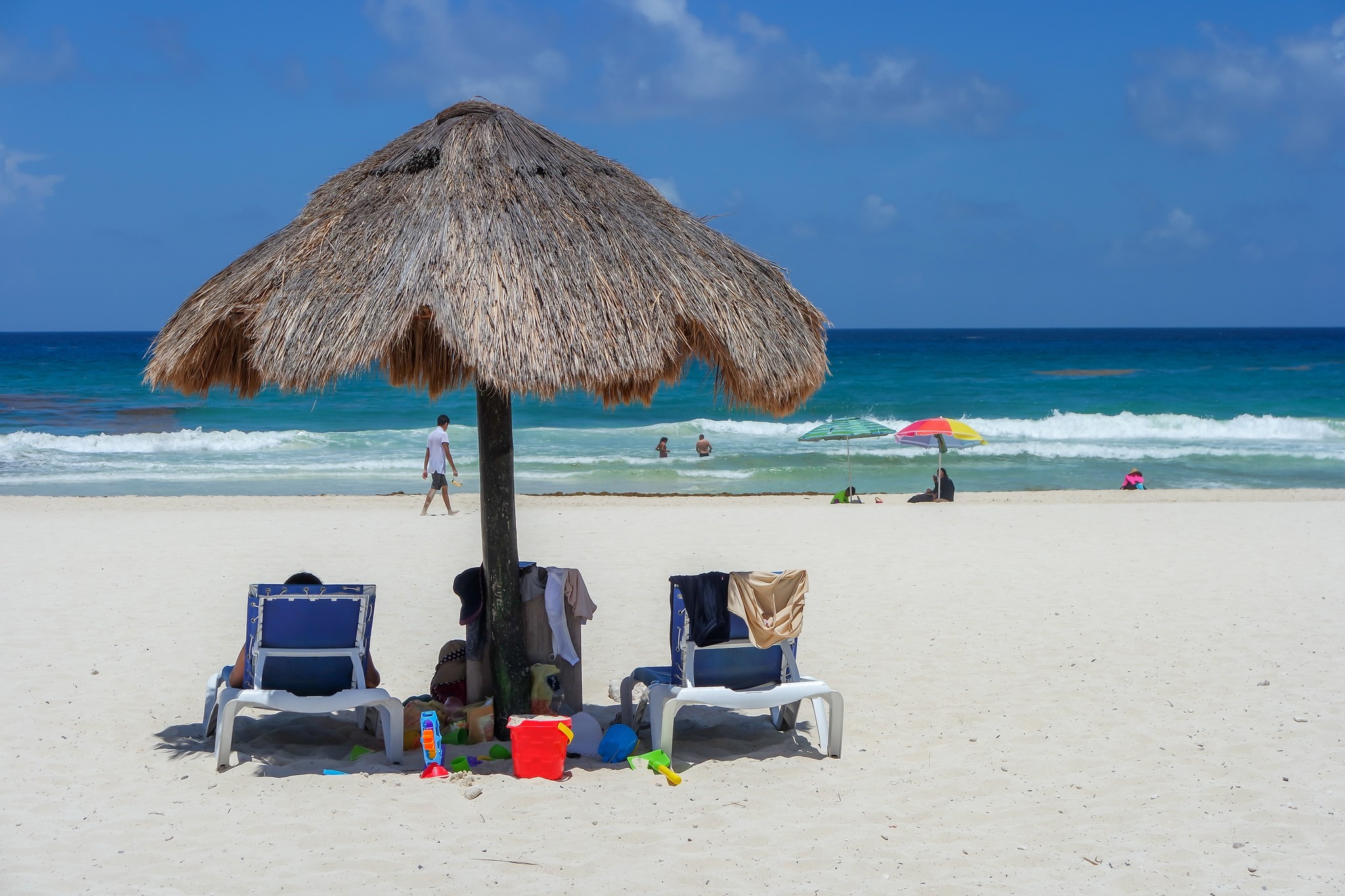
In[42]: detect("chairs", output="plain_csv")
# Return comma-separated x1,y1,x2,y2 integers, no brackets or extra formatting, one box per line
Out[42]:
202,583,405,771
619,567,844,771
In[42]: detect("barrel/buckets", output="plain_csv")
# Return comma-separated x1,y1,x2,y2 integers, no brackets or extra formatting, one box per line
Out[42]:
507,713,574,781
598,724,640,763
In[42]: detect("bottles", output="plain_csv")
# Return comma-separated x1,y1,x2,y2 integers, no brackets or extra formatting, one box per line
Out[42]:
444,692,469,734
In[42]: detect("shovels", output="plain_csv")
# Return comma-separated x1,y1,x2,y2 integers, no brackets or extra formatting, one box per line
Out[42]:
627,749,682,785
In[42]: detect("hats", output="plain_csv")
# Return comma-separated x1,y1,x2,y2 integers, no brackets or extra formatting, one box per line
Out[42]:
430,639,466,689
453,561,484,627
1128,468,1142,476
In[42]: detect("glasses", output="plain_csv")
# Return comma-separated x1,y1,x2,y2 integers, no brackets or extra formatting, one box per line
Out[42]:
452,475,462,486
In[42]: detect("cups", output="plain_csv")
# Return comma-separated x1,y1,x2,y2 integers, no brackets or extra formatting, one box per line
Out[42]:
441,728,467,746
450,756,472,773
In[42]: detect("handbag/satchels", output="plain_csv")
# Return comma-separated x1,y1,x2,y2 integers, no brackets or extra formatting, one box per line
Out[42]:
875,497,883,503
530,662,566,714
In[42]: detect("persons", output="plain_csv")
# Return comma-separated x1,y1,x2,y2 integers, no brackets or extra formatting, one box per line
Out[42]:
916,468,955,503
655,437,670,457
831,486,855,504
419,414,460,516
696,434,712,457
229,573,381,689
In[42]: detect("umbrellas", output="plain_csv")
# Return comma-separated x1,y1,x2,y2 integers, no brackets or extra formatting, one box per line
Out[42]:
796,416,897,501
893,415,989,499
145,98,829,740
